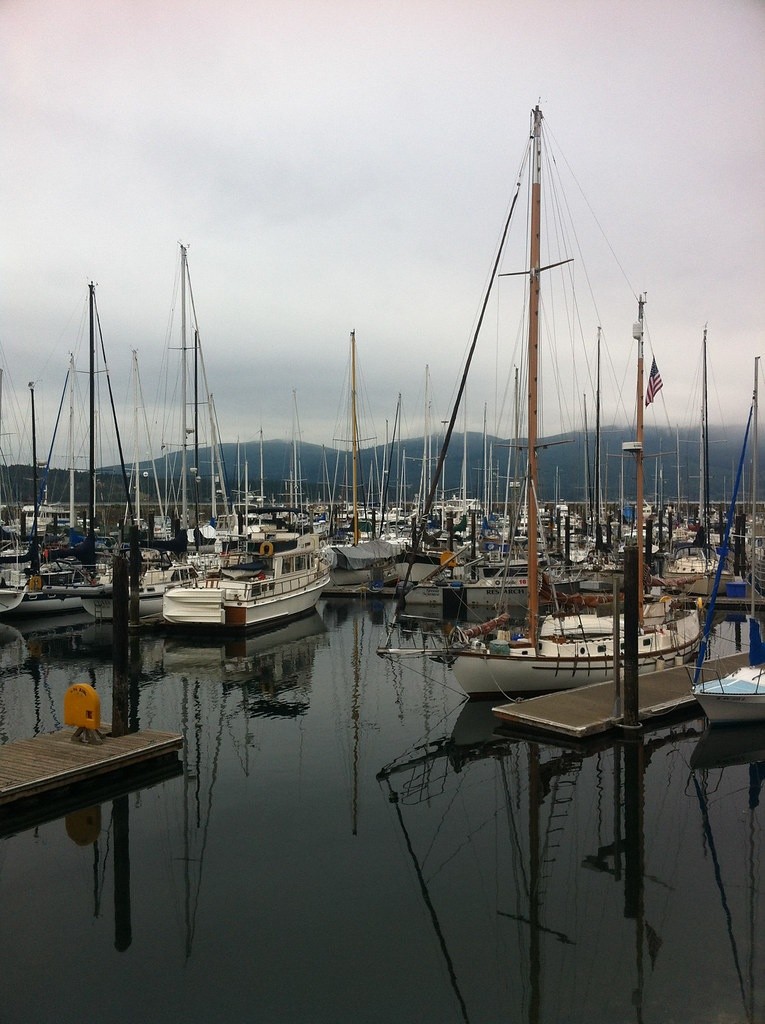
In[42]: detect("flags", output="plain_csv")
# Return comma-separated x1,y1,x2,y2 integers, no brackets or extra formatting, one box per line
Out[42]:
645,355,664,407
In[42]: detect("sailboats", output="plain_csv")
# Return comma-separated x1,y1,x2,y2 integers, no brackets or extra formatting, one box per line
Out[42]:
0,86,765,743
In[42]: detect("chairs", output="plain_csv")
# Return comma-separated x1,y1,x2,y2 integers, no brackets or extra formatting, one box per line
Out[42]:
206,569,223,588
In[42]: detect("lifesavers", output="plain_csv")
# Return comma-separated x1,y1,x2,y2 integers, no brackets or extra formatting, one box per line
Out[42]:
696,596,704,610
259,540,274,558
659,596,674,605
27,574,43,591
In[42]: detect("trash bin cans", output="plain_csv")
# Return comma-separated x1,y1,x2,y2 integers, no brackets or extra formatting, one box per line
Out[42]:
725,581,747,598
